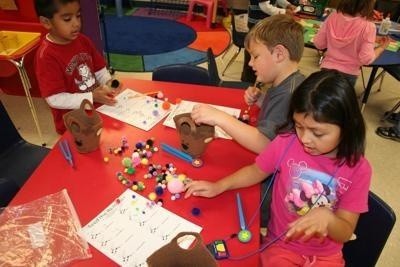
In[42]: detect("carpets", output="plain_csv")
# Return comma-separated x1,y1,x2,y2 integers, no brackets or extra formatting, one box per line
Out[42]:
100,6,232,73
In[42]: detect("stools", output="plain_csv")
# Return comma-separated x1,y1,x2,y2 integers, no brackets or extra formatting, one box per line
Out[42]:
188,0,228,28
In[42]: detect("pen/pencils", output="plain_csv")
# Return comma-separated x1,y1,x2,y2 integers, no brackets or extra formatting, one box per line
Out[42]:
236,192,251,242
161,142,203,168
128,91,158,98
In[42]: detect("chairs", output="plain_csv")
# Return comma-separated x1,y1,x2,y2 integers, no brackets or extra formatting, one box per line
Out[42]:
0,101,52,208
342,190,397,266
153,65,210,85
220,8,249,76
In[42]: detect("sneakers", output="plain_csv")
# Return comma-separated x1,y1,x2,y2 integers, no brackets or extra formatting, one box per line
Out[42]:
376,111,400,141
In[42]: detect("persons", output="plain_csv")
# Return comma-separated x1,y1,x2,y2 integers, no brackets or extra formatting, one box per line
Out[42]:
192,14,307,227
182,70,373,267
241,1,297,34
32,0,119,136
312,0,391,90
376,110,400,142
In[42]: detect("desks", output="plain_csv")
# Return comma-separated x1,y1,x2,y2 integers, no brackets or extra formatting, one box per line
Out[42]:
290,13,400,120
0,31,46,145
0,75,262,266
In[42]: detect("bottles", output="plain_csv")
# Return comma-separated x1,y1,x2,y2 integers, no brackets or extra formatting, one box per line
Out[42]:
379,13,391,35
